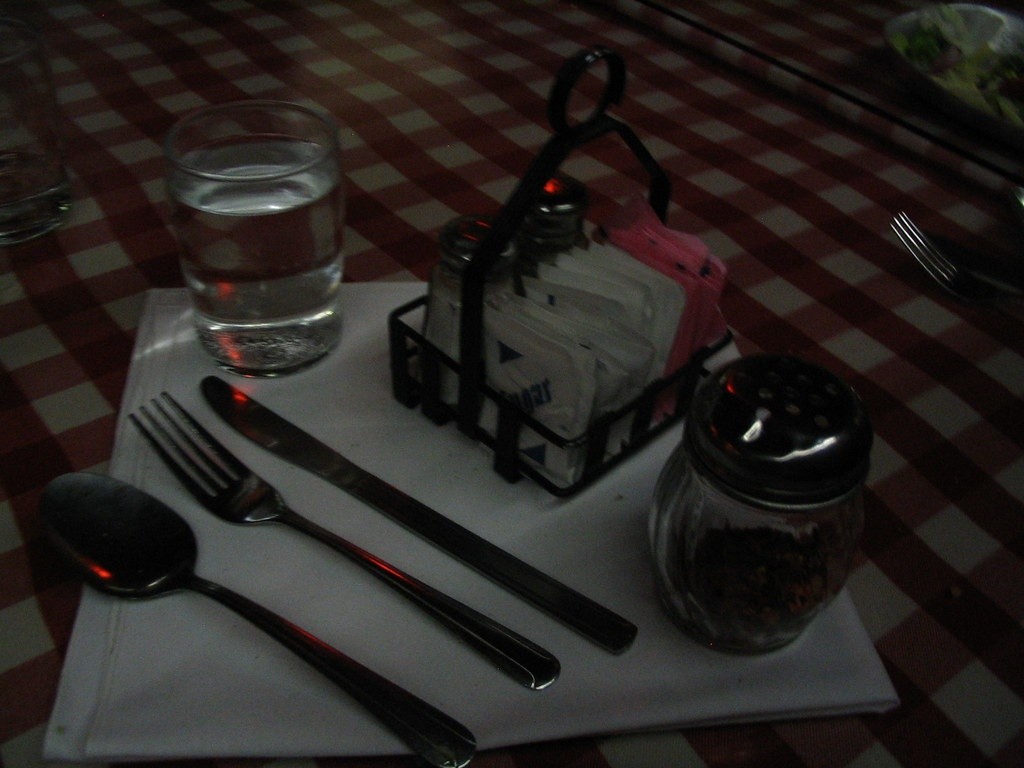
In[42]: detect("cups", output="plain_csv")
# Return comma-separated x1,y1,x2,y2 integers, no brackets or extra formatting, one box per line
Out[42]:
0,18,85,245
163,99,347,378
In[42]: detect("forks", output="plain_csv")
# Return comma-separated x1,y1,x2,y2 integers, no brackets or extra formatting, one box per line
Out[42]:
128,390,563,692
890,210,1024,300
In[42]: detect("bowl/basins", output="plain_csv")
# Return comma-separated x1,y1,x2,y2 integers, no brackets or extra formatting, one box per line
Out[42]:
883,4,1023,151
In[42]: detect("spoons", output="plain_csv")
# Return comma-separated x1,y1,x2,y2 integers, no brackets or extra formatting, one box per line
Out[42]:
37,472,478,768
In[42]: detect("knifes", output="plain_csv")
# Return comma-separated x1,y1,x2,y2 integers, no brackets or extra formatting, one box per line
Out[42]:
201,375,639,655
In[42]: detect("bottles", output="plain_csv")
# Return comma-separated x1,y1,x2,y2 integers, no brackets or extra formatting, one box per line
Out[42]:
420,214,517,403
646,352,877,656
513,173,591,300
